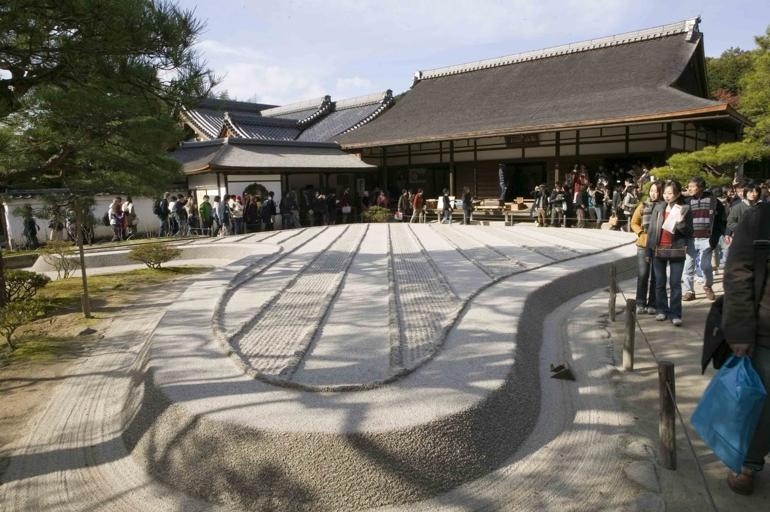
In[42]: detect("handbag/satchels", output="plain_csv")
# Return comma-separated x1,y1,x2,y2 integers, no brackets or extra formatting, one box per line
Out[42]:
342,203,350,213
393,212,403,220
609,211,618,225
154,199,161,214
103,212,110,226
701,295,729,374
654,244,686,259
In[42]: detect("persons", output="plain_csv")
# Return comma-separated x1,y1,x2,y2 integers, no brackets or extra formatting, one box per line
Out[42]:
156,191,276,238
279,184,354,228
441,188,453,224
108,196,137,242
462,186,474,225
630,169,770,329
47,198,94,242
533,165,649,229
722,199,769,497
360,187,425,224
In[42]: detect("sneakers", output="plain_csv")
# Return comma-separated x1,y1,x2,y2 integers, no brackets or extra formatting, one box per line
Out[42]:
648,307,656,314
727,467,755,495
655,314,666,321
703,286,715,300
672,319,682,325
636,307,645,315
683,292,694,301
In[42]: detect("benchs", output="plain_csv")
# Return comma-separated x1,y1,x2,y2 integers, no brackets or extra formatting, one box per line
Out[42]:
407,195,566,224
